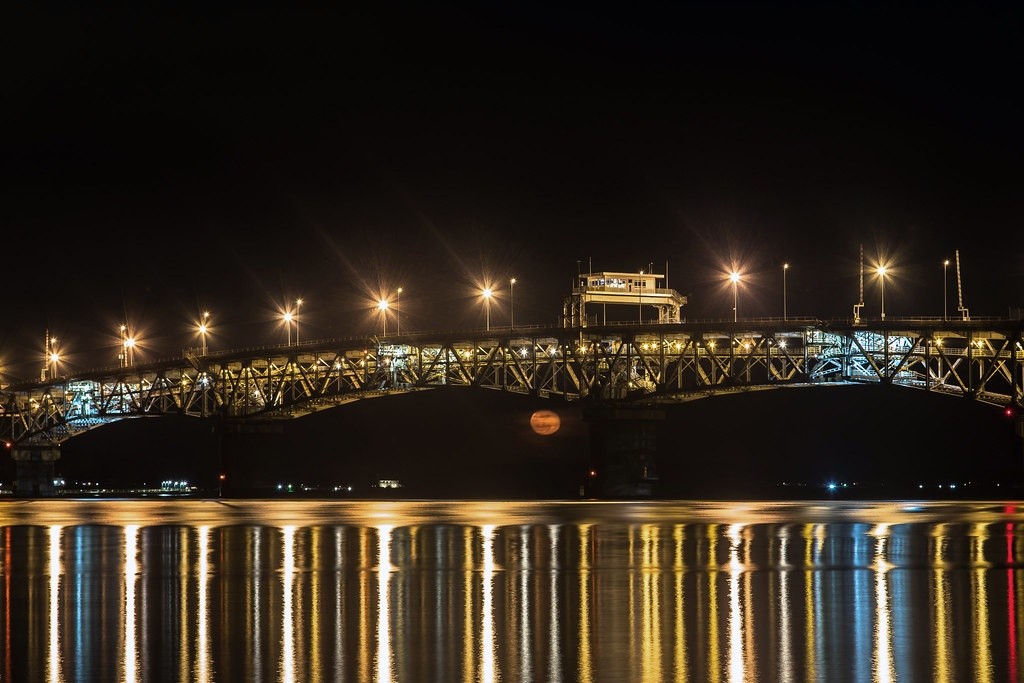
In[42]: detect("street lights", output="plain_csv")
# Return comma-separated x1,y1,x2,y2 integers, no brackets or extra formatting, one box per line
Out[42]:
944,261,949,321
784,263,789,319
878,267,885,321
53,356,57,378
297,300,301,345
484,290,490,331
120,326,125,367
380,301,387,336
640,271,643,323
398,288,402,335
201,327,206,356
129,341,133,366
510,279,515,329
731,274,738,322
285,315,290,346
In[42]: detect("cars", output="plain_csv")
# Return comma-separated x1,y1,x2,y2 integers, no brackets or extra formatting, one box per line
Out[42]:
328,481,353,496
291,480,320,495
